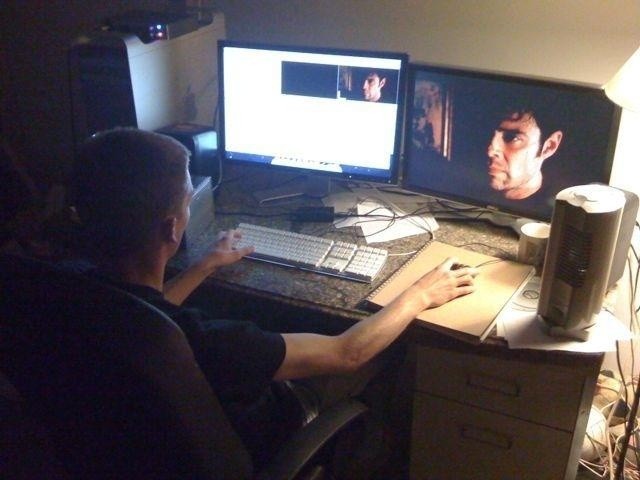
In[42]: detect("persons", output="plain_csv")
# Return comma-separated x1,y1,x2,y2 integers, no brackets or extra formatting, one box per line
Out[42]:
67,127,479,480
358,68,388,102
476,86,564,218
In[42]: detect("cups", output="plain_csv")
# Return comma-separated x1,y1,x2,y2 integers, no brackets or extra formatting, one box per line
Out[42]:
517,222,551,265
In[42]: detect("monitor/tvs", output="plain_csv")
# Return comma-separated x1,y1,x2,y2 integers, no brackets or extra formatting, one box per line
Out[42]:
400,60,623,225
217,39,409,208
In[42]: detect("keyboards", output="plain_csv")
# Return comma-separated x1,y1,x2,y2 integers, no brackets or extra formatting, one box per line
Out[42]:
227,221,389,284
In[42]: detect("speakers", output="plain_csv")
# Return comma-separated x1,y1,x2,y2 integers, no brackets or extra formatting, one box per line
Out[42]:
589,182,640,294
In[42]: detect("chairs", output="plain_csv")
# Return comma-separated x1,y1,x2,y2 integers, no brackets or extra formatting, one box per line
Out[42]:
2,253,371,480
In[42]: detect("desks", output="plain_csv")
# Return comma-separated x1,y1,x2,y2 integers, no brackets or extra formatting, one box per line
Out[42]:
162,163,607,478
